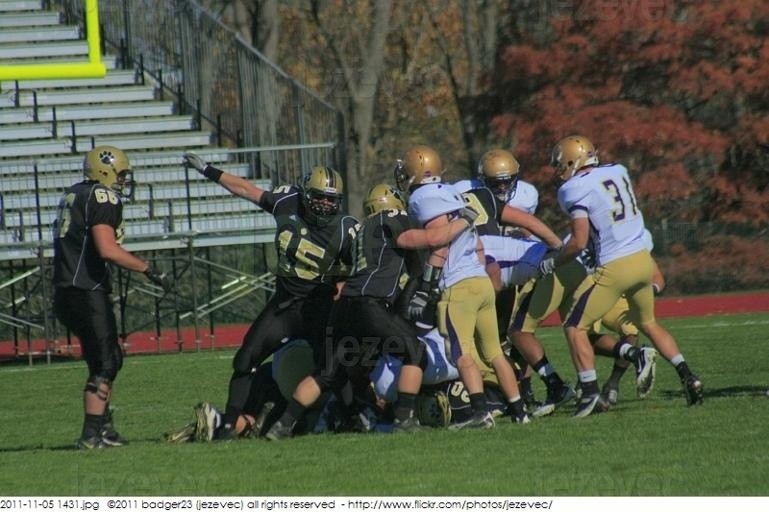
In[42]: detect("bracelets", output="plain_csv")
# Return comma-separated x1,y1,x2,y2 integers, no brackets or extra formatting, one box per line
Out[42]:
539,258,555,279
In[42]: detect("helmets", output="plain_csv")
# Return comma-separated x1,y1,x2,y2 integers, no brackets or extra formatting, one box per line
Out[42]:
82,145,132,197
477,148,521,205
302,166,345,216
394,145,443,194
362,183,408,222
549,135,599,189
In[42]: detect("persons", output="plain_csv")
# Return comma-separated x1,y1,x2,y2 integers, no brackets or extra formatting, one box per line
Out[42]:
52,146,172,449
537,134,704,421
393,146,531,430
452,150,542,342
183,151,363,439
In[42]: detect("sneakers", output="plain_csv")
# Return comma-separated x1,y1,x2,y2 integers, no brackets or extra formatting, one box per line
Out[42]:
95,423,131,447
265,420,303,446
572,382,620,419
76,436,113,451
194,402,220,446
634,345,658,401
681,374,705,408
446,410,497,432
518,380,577,418
510,407,532,426
391,408,434,436
161,421,206,446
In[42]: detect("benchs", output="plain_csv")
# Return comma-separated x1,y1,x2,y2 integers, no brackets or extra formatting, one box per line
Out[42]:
0,0,277,262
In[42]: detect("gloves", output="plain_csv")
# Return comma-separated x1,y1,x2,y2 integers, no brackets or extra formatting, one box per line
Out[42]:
579,246,598,270
539,251,557,277
181,151,210,173
407,289,431,319
458,205,481,225
144,266,171,295
